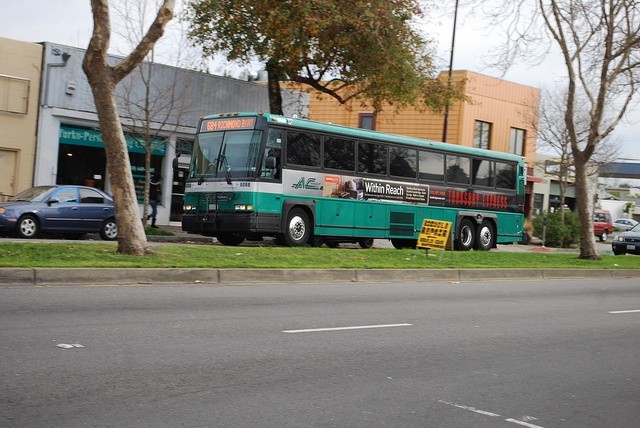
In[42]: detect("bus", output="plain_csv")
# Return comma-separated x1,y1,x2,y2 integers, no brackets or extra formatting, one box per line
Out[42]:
182,112,524,250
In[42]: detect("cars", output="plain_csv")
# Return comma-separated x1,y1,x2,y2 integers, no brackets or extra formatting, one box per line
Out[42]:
0,185,118,241
612,223,640,255
613,219,640,231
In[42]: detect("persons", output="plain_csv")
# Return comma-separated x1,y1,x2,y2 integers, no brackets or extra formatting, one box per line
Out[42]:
140,173,160,229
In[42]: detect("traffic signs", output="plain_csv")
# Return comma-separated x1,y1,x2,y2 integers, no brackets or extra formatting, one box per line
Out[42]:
416,219,452,250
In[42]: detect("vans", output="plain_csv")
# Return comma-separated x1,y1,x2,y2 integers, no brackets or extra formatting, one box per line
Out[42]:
593,209,612,241
